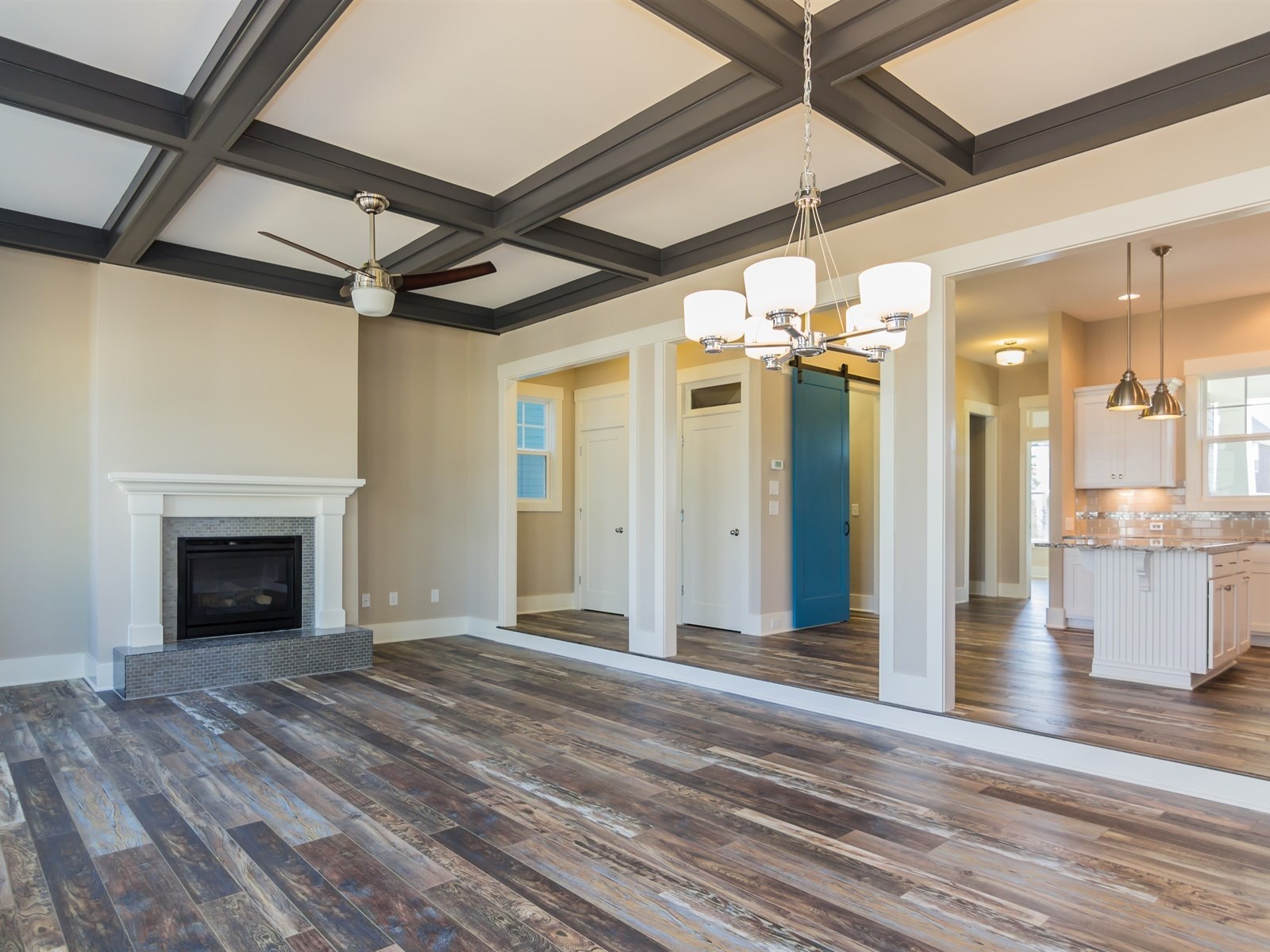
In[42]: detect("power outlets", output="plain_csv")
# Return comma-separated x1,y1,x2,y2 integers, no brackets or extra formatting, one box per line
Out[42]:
1149,523,1163,530
1150,538,1163,546
362,594,370,607
389,592,398,606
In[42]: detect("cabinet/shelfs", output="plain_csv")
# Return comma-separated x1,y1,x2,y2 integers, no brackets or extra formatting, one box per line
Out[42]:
1249,544,1270,647
1073,377,1184,489
1062,548,1094,629
1089,549,1251,690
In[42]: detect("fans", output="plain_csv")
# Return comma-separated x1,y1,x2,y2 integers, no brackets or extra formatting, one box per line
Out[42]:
257,192,497,290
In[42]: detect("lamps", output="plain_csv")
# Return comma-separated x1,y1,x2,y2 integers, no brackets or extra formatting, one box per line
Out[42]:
1105,242,1153,411
1136,246,1186,420
995,341,1027,367
682,1,933,369
352,282,396,317
